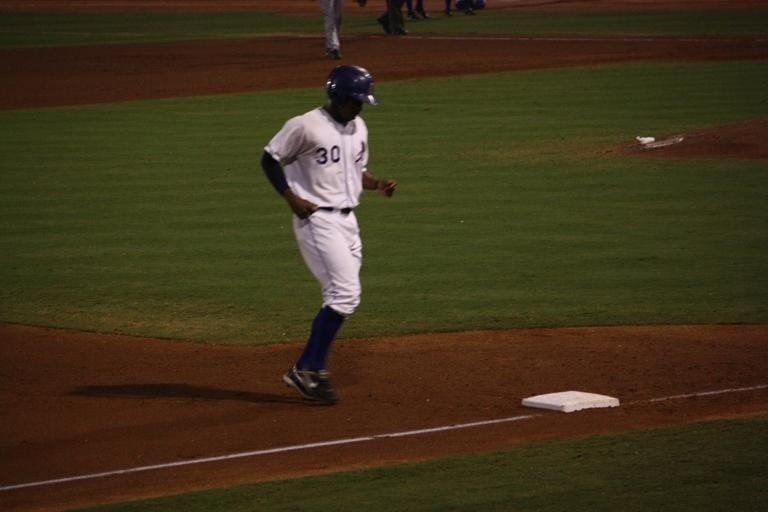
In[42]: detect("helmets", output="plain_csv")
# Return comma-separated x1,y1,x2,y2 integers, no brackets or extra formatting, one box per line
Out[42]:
325,64,378,106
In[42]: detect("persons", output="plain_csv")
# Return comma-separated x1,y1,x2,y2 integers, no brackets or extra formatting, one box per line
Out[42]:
320,0,342,59
358,0,367,7
378,0,485,35
261,64,398,406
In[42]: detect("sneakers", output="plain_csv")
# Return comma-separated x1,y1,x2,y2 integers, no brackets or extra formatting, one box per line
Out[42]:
324,48,342,59
464,9,476,15
407,10,432,20
282,363,340,405
377,15,408,35
444,8,453,17
